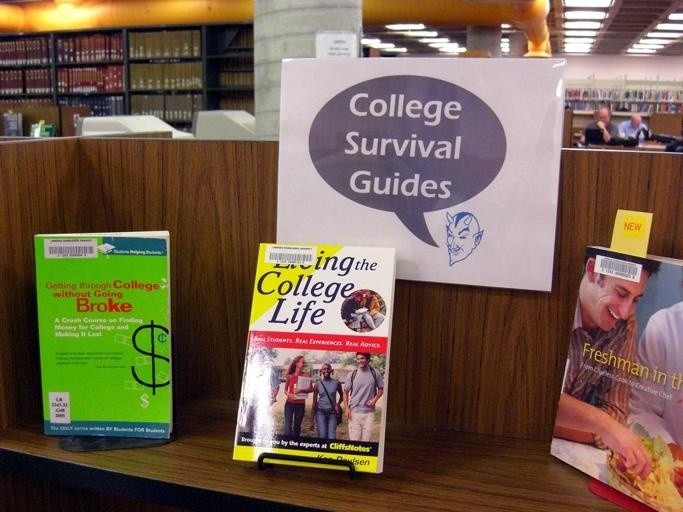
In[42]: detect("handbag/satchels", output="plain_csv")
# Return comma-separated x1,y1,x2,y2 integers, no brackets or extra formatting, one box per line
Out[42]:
336,407,342,424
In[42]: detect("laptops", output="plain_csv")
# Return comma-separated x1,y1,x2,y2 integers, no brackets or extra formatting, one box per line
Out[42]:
585,128,604,145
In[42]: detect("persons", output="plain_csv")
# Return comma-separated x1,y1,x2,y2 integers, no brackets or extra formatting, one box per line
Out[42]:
446,211,484,266
573,107,618,146
552,246,661,480
342,352,384,442
342,291,362,332
626,301,683,448
310,363,344,440
617,114,648,144
360,291,384,329
244,365,281,433
283,356,314,434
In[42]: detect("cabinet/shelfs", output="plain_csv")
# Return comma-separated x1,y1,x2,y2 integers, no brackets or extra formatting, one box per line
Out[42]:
563,72,682,117
204,23,254,115
0,31,56,139
126,23,205,133
51,27,126,133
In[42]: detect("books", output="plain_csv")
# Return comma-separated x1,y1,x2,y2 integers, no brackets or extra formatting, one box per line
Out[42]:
564,88,683,113
33,230,175,440
0,29,255,123
232,242,396,473
550,246,683,512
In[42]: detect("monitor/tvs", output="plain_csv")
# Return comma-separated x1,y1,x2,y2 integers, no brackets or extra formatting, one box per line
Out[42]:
74,115,196,140
192,111,255,140
